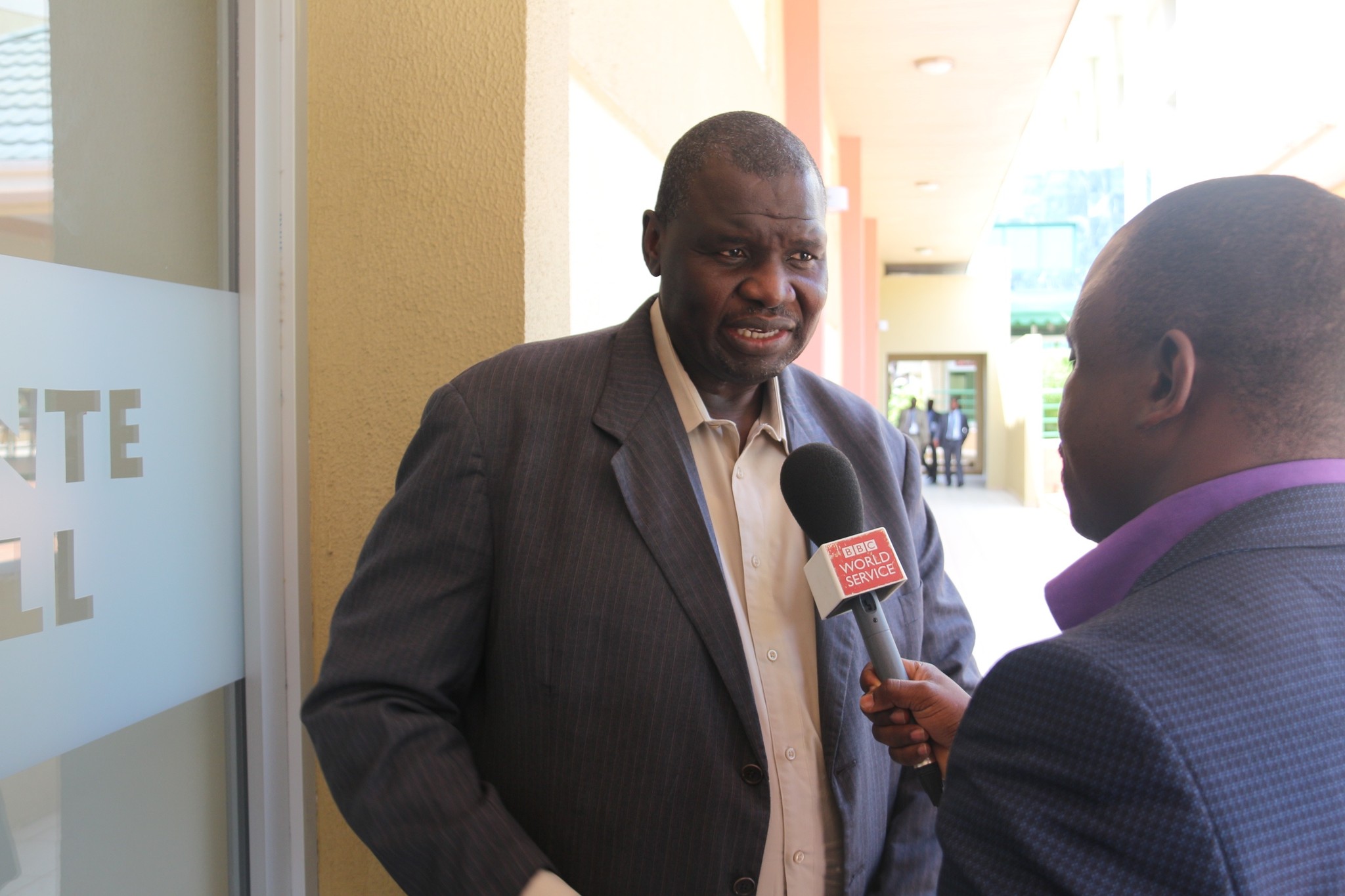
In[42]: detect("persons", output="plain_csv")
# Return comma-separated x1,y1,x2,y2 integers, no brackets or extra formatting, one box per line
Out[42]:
851,172,1344,895
932,395,969,487
895,396,930,465
919,399,944,484
290,111,988,896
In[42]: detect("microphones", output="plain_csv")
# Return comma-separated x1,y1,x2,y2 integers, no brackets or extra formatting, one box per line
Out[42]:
781,442,942,806
